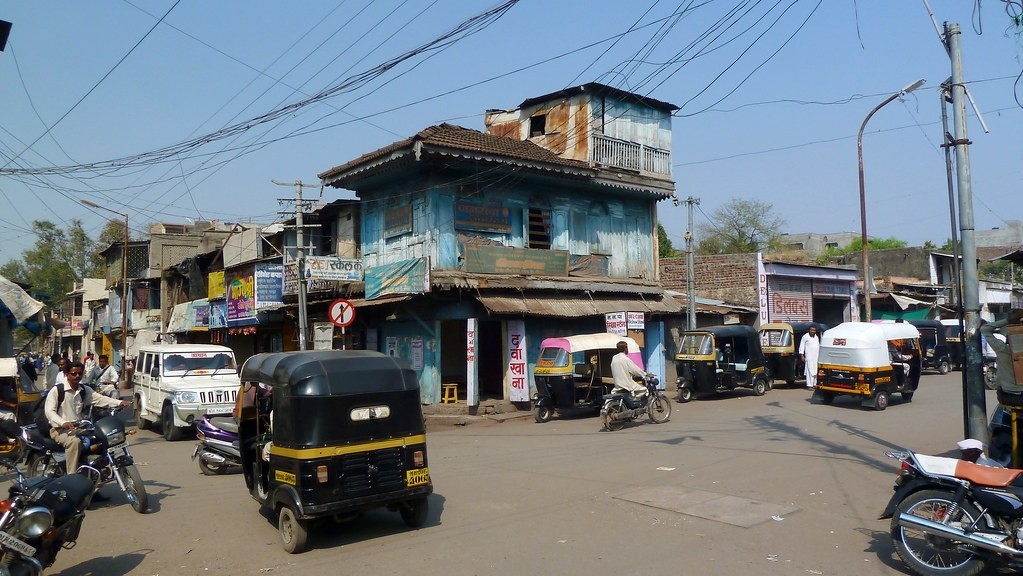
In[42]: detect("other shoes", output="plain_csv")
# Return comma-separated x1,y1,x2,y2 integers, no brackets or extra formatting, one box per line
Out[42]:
806,387,814,391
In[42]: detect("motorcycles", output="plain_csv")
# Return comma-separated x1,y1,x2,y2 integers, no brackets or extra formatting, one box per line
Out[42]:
675,325,767,403
81,382,124,419
908,320,952,374
876,447,1023,576
939,319,967,370
0,456,100,576
814,322,922,411
600,373,672,431
240,349,433,554
19,402,148,513
532,332,644,423
756,322,828,391
981,327,1007,390
0,357,47,467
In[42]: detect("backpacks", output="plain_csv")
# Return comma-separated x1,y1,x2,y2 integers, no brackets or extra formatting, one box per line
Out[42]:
33,382,86,437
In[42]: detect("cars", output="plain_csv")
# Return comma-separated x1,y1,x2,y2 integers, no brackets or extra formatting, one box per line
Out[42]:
20,355,28,362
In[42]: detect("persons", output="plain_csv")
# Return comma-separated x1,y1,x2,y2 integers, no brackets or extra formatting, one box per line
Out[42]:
799,325,820,390
15,349,120,416
888,341,914,390
44,362,131,475
257,409,274,500
980,306,1023,407
611,341,654,400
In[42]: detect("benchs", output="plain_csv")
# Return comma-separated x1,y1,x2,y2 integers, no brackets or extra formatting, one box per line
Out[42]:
717,359,749,376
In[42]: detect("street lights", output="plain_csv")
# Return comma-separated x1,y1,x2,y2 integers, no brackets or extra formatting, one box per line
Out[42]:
81,199,128,389
859,78,927,323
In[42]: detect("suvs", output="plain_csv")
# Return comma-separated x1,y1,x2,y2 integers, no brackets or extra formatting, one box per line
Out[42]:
133,344,241,441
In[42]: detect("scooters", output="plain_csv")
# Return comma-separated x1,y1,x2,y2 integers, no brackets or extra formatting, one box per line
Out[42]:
190,414,241,475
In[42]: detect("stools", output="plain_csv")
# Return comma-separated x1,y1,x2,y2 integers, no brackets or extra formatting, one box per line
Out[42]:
442,384,458,404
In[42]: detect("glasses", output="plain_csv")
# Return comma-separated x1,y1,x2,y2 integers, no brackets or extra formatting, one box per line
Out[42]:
68,371,83,376
